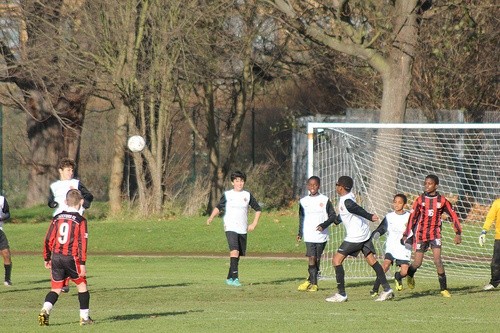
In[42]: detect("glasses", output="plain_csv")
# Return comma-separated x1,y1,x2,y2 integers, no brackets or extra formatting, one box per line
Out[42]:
336,182,340,186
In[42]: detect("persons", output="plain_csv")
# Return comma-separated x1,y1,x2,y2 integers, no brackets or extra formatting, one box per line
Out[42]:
478,197,500,291
37,188,95,326
296,175,336,290
324,176,394,302
47,159,93,293
400,174,461,297
0,196,13,286
368,194,414,296
207,170,261,287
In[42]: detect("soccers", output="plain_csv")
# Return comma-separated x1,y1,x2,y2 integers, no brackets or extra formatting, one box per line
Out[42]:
128,135,145,152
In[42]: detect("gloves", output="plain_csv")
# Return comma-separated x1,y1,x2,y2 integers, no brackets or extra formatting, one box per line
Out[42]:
479,231,487,246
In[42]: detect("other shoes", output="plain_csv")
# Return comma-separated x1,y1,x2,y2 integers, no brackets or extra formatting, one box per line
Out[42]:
483,283,494,290
62,286,69,292
4,280,13,285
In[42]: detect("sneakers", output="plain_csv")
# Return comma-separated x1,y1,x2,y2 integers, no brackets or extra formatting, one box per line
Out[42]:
225,277,242,286
366,290,378,297
406,275,415,289
394,272,403,291
440,289,451,298
38,311,50,326
375,289,394,301
298,278,318,291
81,316,94,325
325,293,347,302
